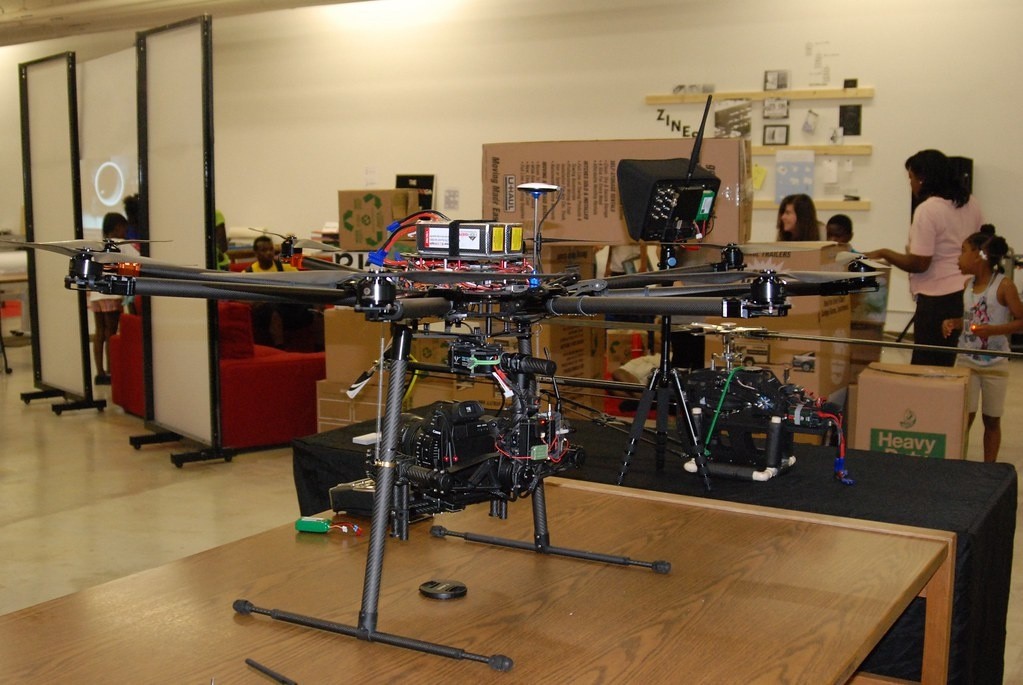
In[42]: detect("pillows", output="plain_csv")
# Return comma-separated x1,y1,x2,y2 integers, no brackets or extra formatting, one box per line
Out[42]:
216,302,252,360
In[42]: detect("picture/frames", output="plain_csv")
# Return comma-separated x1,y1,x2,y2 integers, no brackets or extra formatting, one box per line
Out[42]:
764,71,788,89
763,124,790,145
763,98,788,118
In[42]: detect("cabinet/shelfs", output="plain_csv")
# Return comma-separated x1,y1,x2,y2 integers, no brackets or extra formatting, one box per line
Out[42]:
644,87,873,211
0,235,31,347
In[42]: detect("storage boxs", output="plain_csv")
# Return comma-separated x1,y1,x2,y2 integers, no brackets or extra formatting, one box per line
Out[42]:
855,361,970,459
703,241,850,417
338,189,420,251
316,242,605,435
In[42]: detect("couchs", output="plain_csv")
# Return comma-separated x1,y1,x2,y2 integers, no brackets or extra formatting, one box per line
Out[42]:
109,314,316,448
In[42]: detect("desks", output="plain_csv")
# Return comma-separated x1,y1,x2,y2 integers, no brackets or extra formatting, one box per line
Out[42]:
0,476,956,685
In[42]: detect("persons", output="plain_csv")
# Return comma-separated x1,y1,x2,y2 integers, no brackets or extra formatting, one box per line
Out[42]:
824,214,861,254
210,211,232,270
90,212,142,386
121,194,142,255
775,193,827,242
238,236,314,350
941,224,1023,465
859,146,982,369
